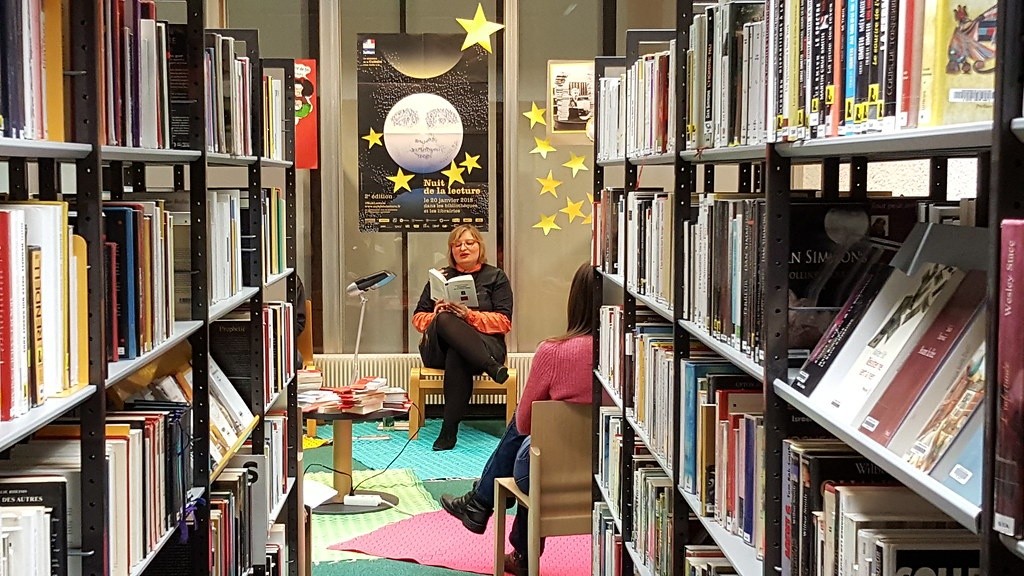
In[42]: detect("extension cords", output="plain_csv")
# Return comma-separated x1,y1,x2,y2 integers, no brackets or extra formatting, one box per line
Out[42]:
344,494,384,507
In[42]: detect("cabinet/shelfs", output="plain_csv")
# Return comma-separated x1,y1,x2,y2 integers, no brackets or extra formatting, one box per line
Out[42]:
592,0,1024,576
0,0,295,576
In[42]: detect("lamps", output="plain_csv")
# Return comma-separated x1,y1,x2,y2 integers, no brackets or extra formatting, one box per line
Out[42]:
348,269,398,383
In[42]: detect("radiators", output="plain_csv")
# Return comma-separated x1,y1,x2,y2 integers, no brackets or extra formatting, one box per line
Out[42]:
312,352,535,405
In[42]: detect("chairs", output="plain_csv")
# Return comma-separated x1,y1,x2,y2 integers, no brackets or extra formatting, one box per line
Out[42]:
408,336,518,441
493,401,593,576
297,299,313,362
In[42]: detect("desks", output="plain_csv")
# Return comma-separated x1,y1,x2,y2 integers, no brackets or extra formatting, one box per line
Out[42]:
301,407,412,515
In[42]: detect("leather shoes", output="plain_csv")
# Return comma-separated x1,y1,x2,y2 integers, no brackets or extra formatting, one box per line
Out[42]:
440,480,494,534
504,548,528,576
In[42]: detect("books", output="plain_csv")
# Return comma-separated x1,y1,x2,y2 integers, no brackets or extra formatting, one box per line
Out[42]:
428,268,478,307
0,398,192,576
297,370,412,415
789,252,987,508
590,0,998,161
126,300,294,476
142,410,290,576
0,0,193,150
203,32,284,160
590,186,931,373
103,198,175,363
591,304,981,576
174,187,285,314
0,199,79,423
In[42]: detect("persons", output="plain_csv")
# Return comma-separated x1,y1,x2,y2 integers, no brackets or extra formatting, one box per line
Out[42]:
440,258,592,576
297,274,307,367
411,225,513,451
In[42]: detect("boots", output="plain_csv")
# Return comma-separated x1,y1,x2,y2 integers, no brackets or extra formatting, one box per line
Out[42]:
433,419,458,449
485,355,509,384
